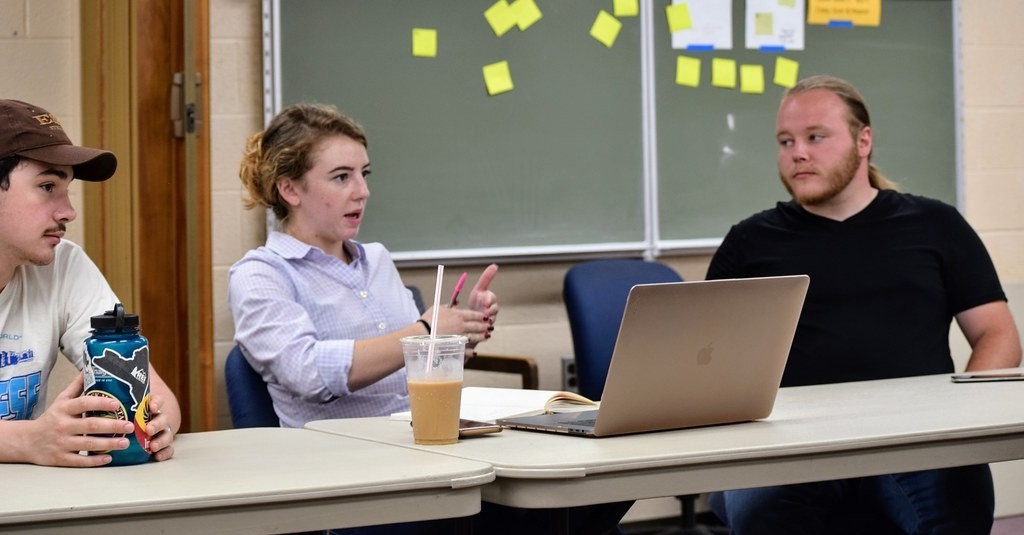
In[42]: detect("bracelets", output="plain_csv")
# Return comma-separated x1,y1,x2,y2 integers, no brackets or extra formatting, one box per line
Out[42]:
416,319,431,332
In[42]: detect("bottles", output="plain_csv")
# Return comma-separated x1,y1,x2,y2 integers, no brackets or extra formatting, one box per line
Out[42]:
83,306,150,464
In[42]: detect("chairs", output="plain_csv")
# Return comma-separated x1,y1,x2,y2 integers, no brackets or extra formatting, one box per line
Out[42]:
562,259,735,535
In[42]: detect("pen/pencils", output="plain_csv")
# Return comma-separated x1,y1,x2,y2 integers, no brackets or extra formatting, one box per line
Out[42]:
447,271,467,307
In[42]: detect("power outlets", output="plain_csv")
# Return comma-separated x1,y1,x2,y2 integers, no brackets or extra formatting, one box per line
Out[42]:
562,357,577,391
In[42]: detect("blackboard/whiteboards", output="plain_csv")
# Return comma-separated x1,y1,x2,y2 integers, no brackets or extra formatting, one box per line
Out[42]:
260,0,966,270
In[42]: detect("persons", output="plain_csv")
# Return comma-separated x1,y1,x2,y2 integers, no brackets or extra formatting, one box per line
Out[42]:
704,76,1021,535
227,103,498,430
0,100,181,468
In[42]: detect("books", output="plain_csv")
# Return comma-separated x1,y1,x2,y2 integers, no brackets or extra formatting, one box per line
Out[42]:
391,387,599,422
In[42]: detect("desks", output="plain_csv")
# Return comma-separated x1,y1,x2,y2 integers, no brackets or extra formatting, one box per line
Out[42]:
304,369,1024,535
0,428,494,535
226,340,540,430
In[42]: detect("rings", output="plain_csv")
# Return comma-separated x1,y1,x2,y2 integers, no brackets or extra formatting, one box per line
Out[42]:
169,426,172,432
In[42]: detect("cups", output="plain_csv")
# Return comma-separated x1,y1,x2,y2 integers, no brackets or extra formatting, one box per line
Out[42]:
401,334,469,443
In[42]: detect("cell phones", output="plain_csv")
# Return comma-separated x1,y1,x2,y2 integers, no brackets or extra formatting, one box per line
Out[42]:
951,373,1024,383
411,419,503,437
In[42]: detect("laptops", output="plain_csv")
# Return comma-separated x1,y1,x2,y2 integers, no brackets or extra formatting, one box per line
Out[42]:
496,274,811,437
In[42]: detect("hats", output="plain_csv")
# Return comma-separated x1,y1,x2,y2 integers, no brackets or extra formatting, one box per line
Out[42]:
0,99,117,182
